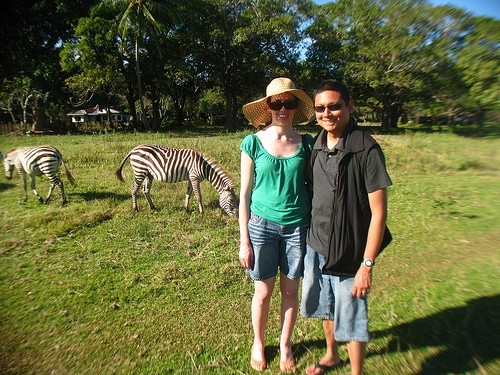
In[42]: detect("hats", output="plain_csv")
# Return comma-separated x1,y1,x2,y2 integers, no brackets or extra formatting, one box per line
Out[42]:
243,78,315,127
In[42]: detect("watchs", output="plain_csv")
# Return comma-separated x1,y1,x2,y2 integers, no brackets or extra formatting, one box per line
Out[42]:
361,258,375,267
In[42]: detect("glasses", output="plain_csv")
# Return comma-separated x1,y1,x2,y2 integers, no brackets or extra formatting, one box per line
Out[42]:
267,100,299,110
315,103,343,113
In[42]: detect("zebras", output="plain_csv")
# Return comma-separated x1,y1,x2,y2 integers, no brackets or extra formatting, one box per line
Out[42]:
114,143,238,219
0,145,78,206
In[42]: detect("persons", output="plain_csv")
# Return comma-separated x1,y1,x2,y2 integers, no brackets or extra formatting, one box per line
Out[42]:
299,80,394,374
238,78,317,375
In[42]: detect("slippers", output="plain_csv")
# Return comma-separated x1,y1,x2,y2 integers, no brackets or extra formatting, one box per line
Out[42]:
280,358,294,373
305,357,342,375
249,357,267,372
